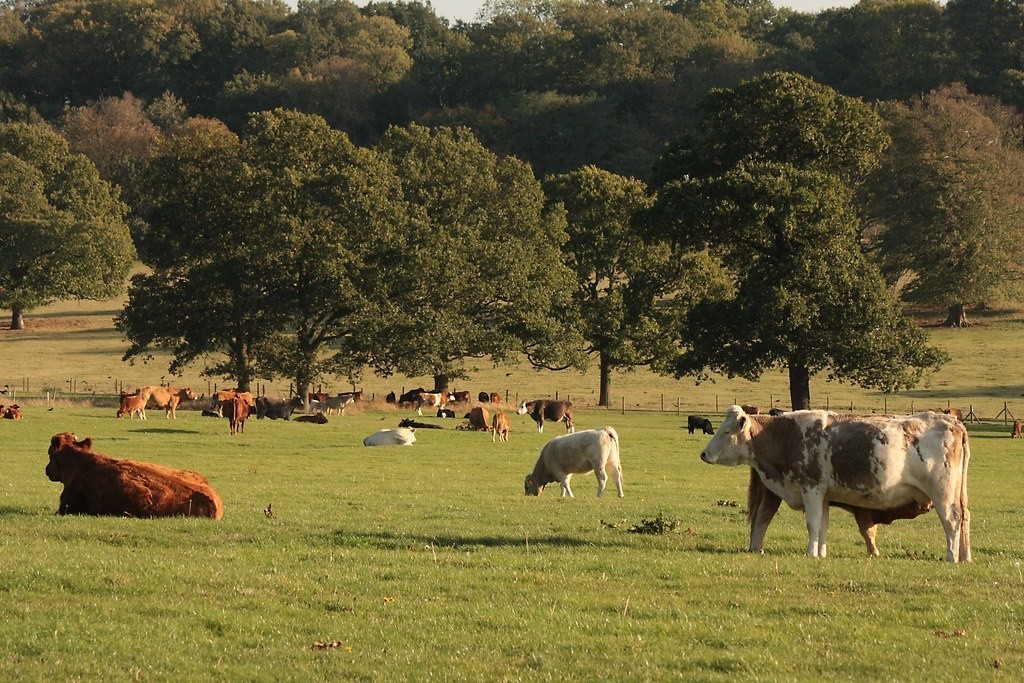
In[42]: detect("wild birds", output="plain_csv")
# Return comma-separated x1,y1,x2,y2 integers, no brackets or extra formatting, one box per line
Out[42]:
65,376,113,392
264,503,275,519
0,384,9,395
48,408,53,411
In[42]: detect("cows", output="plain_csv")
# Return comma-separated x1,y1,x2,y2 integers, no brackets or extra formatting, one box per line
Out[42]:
688,415,714,435
45,432,218,519
116,386,363,436
1011,419,1024,439
437,409,455,419
524,426,624,498
363,426,417,447
0,404,23,420
386,387,455,416
490,392,500,405
479,391,489,403
700,403,971,563
399,418,440,430
450,391,471,407
491,412,510,442
517,398,575,434
468,406,490,432
